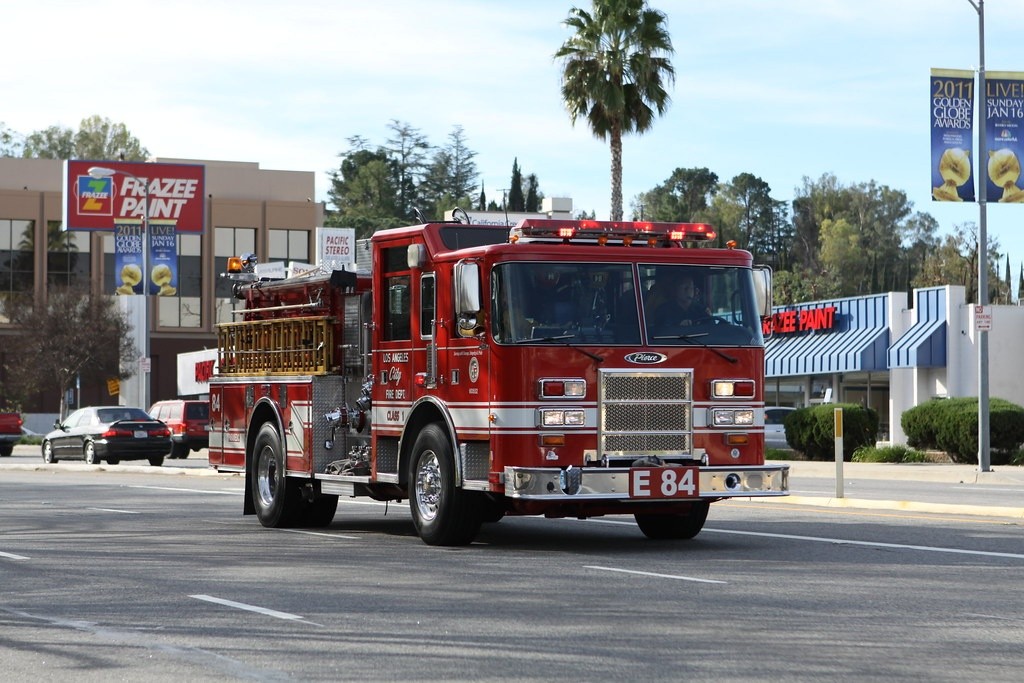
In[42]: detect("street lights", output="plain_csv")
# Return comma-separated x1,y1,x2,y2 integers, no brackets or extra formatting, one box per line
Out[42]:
87,167,151,411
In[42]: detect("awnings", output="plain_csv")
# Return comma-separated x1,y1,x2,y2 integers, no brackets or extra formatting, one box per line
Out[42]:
764,322,947,379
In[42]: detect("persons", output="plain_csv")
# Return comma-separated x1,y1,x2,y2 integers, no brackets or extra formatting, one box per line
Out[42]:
653,275,716,331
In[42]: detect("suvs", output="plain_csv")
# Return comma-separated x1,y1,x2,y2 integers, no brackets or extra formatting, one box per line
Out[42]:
146,400,209,458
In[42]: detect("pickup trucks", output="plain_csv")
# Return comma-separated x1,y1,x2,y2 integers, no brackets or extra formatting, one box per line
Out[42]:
0,413,23,457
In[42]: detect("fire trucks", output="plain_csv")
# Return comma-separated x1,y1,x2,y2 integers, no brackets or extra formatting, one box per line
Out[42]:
205,197,788,547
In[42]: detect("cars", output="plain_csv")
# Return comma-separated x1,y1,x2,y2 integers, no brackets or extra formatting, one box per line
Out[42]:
763,407,793,450
42,406,171,465
